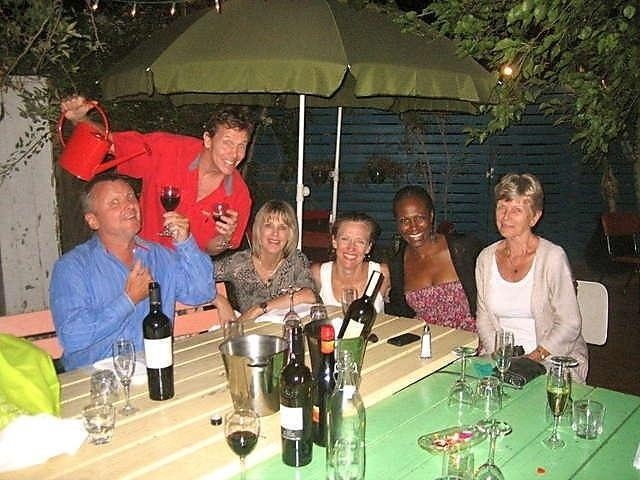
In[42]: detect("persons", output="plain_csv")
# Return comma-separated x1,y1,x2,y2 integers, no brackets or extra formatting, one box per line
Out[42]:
383,184,484,331
212,201,317,328
60,91,256,258
311,212,390,318
49,173,215,373
469,173,590,384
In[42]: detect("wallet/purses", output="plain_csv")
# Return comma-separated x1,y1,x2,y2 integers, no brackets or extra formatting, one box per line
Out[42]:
493,355,546,387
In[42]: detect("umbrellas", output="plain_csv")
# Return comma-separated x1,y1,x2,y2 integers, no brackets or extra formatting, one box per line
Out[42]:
97,0,503,251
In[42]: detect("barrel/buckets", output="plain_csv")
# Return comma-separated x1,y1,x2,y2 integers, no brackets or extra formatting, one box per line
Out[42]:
218,331,291,420
302,318,367,395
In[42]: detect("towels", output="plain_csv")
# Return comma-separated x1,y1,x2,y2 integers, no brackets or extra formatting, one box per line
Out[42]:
503,358,547,387
0,413,87,472
94,356,147,384
255,305,318,325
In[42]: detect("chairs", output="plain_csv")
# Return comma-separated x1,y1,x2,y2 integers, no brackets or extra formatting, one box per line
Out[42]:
598,213,640,294
575,280,609,345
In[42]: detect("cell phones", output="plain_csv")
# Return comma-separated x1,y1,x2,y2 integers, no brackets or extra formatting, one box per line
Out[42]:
385,332,420,347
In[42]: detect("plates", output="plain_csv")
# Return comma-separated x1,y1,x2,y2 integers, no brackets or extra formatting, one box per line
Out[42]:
92,356,150,381
416,423,488,458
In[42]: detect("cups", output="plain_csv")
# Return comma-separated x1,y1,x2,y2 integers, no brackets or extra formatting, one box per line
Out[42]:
81,368,121,446
224,318,244,342
572,398,606,441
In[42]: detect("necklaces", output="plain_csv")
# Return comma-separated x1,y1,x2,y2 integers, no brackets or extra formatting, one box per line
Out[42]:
259,257,281,280
505,251,526,275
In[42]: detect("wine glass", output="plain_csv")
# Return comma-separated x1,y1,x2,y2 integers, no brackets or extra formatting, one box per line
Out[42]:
212,201,235,249
110,336,141,417
224,408,262,480
440,328,579,479
157,183,182,237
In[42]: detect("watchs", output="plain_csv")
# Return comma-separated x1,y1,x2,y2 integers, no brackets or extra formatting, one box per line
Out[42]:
537,349,545,361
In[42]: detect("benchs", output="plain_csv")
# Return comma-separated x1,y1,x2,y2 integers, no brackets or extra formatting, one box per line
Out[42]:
0,281,230,360
245,210,337,255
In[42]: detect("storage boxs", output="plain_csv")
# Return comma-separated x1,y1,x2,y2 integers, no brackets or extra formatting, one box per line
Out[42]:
353,157,436,185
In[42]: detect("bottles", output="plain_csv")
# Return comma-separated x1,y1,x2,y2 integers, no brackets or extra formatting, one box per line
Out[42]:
418,323,433,359
276,270,387,480
140,280,179,402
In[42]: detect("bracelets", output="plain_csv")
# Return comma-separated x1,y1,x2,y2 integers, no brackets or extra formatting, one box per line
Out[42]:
260,302,267,313
